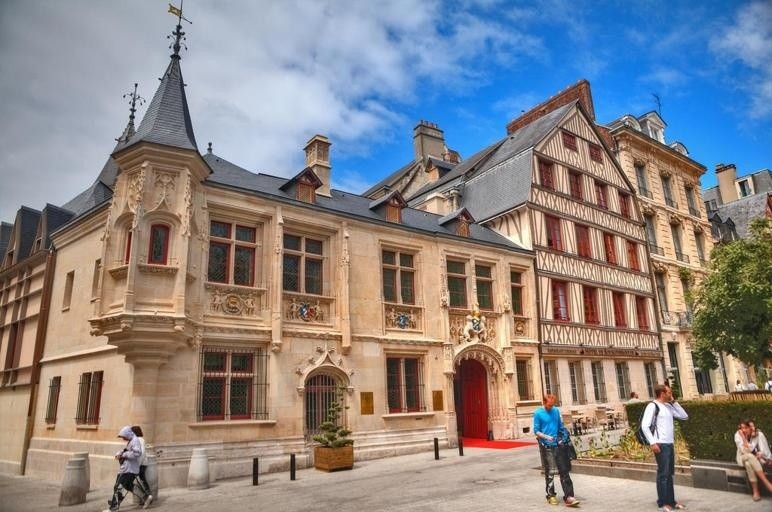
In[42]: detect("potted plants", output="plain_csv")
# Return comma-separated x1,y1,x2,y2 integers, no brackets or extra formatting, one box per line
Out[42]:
313,382,354,471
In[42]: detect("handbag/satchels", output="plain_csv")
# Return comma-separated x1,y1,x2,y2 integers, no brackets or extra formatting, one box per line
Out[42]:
119,448,129,464
635,401,660,445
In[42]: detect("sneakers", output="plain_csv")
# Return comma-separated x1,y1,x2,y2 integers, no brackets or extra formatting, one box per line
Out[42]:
672,502,689,510
139,495,154,509
565,496,579,506
102,499,120,512
547,495,559,505
658,505,673,512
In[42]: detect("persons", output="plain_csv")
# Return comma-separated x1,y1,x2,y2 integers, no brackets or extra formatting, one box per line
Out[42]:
627,392,643,404
733,417,772,501
103,426,155,512
735,376,772,392
642,382,690,512
532,393,580,508
